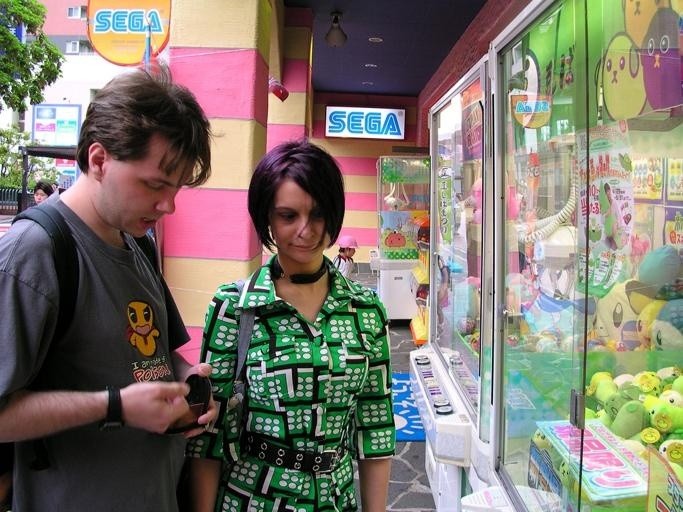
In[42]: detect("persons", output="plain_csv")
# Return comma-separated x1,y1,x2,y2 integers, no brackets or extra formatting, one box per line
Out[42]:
186,143,396,512
34,183,54,205
332,236,358,278
0,73,216,512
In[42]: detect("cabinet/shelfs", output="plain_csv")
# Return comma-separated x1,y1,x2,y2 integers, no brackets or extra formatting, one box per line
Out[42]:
408,54,489,512
378,154,431,347
488,1,681,512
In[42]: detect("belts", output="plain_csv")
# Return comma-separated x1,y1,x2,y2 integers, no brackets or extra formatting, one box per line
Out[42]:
239,432,345,475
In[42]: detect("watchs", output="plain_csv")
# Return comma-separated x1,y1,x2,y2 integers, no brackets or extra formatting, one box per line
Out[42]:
99,387,124,432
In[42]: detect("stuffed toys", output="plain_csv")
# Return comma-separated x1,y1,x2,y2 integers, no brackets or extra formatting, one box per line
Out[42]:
441,176,683,467
440,177,683,466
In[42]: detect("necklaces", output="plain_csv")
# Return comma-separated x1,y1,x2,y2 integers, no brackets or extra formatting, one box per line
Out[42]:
272,255,327,283
